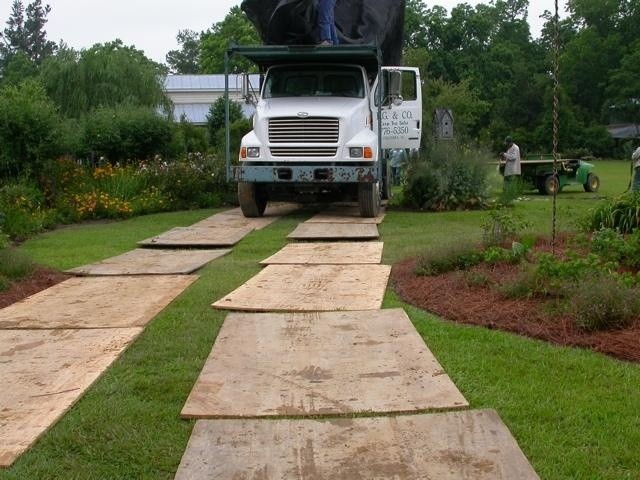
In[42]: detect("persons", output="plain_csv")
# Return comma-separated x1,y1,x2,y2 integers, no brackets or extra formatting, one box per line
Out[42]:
499,137,522,177
315,0,339,48
389,148,404,186
632,140,640,191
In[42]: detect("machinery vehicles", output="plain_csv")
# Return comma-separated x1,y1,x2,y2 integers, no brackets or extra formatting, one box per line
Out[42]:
593,123,640,159
498,153,599,195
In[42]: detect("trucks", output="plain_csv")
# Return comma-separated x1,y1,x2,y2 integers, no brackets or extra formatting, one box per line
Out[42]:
224,43,423,218
